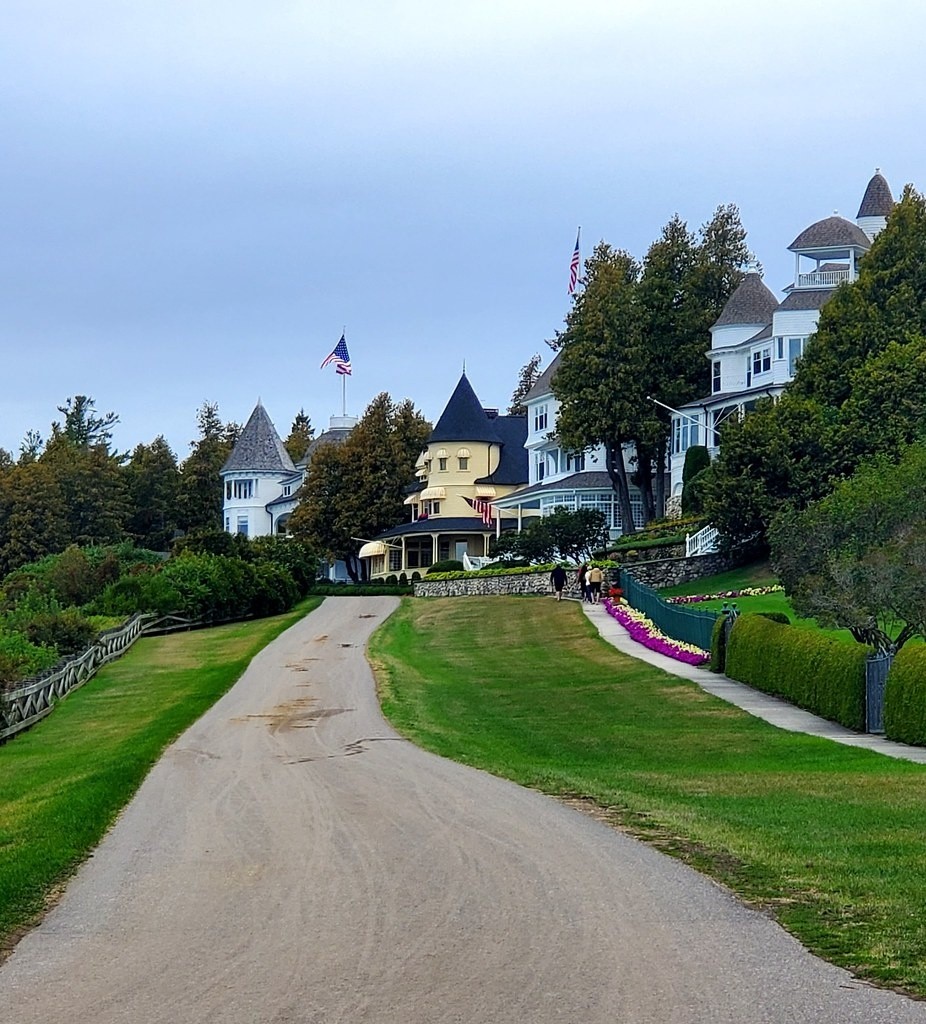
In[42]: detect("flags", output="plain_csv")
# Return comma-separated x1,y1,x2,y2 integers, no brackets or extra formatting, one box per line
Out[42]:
320,334,352,376
567,235,580,295
461,495,493,528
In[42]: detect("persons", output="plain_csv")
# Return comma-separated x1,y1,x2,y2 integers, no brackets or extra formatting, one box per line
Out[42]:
550,564,568,601
578,564,602,605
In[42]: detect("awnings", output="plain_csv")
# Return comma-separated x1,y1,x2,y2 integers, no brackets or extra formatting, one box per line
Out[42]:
415,450,427,468
457,449,470,458
404,495,418,505
359,541,385,559
423,452,432,460
414,469,428,476
437,449,449,459
475,486,496,498
419,487,446,500
